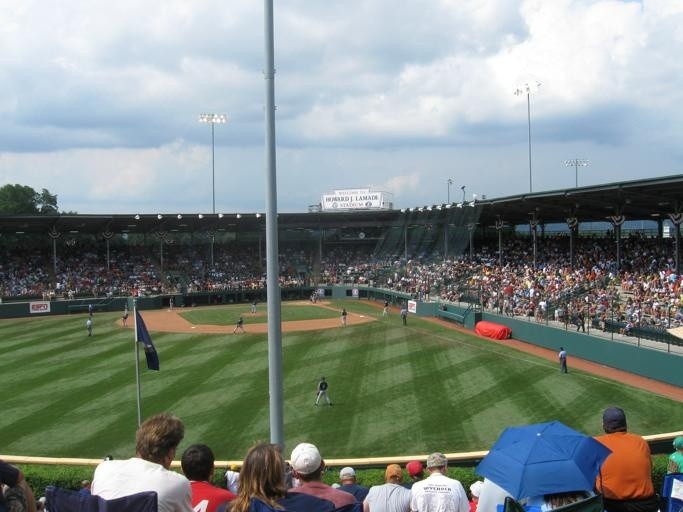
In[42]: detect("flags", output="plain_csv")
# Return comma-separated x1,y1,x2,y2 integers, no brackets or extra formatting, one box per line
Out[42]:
134,307,159,371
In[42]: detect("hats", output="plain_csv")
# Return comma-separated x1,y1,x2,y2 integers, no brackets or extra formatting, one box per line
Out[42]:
339,453,483,498
673,437,683,449
603,407,626,428
291,443,321,474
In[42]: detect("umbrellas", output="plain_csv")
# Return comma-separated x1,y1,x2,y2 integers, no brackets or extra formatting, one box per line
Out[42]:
474,420,613,502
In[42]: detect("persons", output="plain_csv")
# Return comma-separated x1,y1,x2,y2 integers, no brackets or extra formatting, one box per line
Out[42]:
170,296,174,310
592,407,663,512
91,413,196,512
216,444,336,512
0,454,113,512
315,377,332,406
332,452,484,512
233,317,246,336
558,347,567,373
225,464,241,495
122,306,128,327
252,300,256,313
277,232,682,336
286,442,358,512
526,490,597,512
181,444,238,512
284,462,300,490
667,436,683,475
86,303,93,336
1,232,266,302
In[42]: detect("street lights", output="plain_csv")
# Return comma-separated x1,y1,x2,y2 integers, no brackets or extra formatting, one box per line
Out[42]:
562,158,590,188
197,111,229,214
446,179,452,204
512,78,546,193
461,185,466,203
